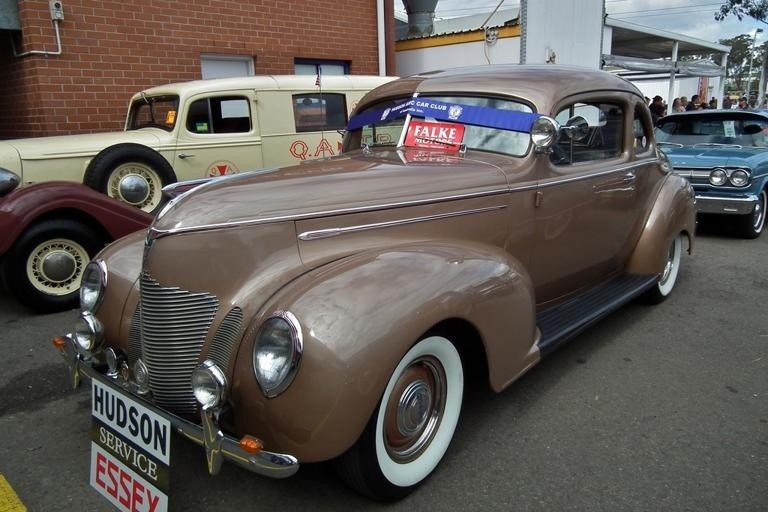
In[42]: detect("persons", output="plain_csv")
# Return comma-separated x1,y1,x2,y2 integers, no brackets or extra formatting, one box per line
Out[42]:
644,95,750,126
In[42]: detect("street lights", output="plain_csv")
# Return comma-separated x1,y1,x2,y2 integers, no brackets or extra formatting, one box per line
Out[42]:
746,28,763,102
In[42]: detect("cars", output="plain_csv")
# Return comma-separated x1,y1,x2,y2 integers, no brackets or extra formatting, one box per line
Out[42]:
52,64,698,504
0,74,402,311
653,108,768,238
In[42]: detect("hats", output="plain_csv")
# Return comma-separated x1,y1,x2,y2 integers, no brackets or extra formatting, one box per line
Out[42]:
738,96,747,101
